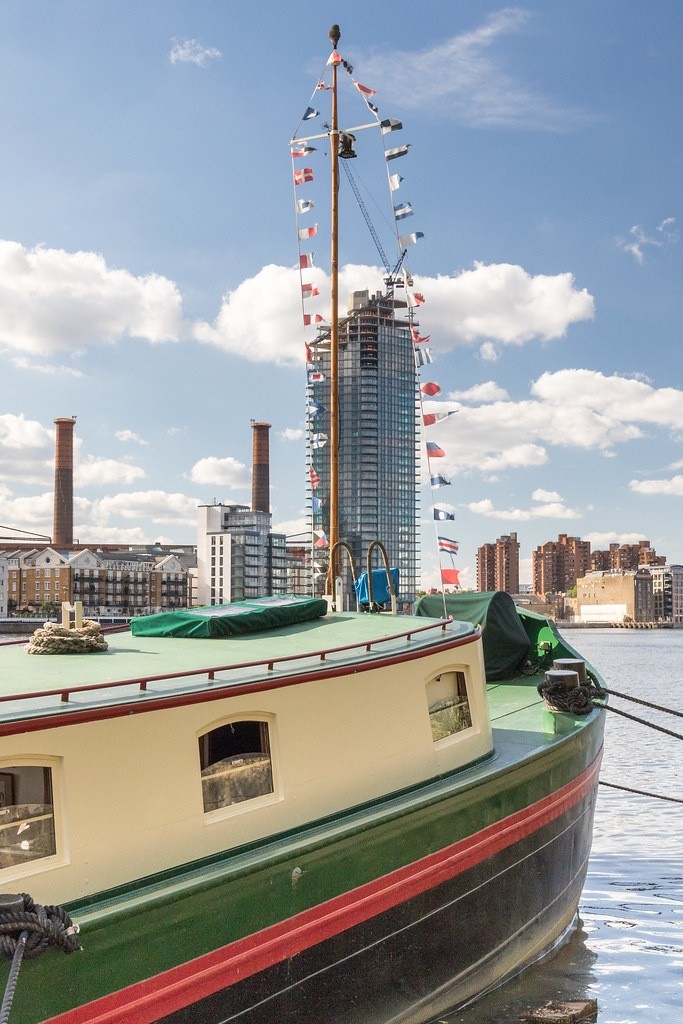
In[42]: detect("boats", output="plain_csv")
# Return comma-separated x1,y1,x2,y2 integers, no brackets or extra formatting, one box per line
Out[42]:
0,25,609,1024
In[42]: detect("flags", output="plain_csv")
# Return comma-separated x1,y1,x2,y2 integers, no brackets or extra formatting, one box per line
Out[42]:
290,48,462,585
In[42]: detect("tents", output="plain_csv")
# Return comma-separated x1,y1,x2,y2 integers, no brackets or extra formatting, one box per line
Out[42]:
412,591,535,682
